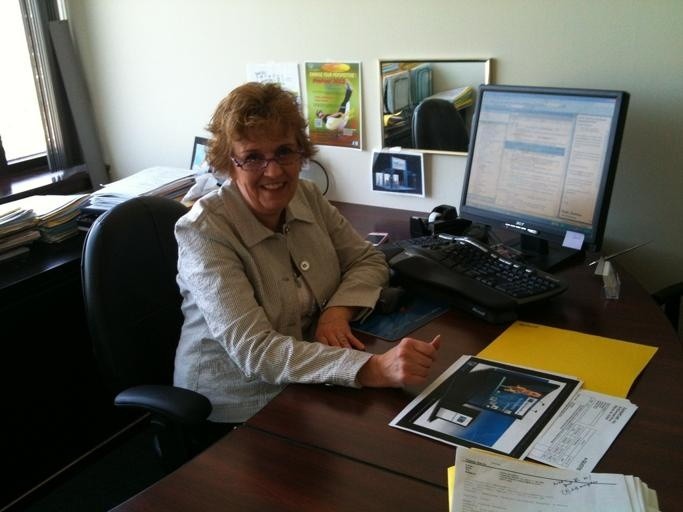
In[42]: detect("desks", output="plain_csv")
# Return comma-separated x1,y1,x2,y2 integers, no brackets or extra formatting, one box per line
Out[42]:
384,97,473,140
109,192,683,512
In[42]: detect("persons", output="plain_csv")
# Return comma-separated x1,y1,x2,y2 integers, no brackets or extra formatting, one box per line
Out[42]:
316,80,353,123
173,81,444,440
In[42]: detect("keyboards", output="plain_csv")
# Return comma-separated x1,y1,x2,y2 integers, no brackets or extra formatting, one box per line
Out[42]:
380,233,570,324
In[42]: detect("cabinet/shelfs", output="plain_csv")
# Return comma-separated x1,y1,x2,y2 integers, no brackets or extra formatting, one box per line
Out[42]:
1,222,151,511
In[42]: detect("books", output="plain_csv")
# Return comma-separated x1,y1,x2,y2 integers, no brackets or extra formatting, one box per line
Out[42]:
446,445,660,512
382,86,474,142
0,166,196,264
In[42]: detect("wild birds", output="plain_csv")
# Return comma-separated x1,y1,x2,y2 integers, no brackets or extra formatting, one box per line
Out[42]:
317,80,352,138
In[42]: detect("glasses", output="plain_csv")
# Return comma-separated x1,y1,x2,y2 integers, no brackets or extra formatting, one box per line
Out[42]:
232,153,299,170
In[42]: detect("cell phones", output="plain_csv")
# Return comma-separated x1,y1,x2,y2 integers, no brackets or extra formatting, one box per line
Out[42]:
364,230,392,247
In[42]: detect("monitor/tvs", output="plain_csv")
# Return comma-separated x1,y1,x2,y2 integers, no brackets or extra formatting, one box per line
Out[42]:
457,82,631,273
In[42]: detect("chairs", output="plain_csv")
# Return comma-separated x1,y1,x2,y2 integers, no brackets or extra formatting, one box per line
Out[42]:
410,97,470,152
80,196,213,463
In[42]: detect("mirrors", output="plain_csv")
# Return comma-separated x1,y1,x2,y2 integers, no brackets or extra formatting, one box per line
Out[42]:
379,57,492,155
